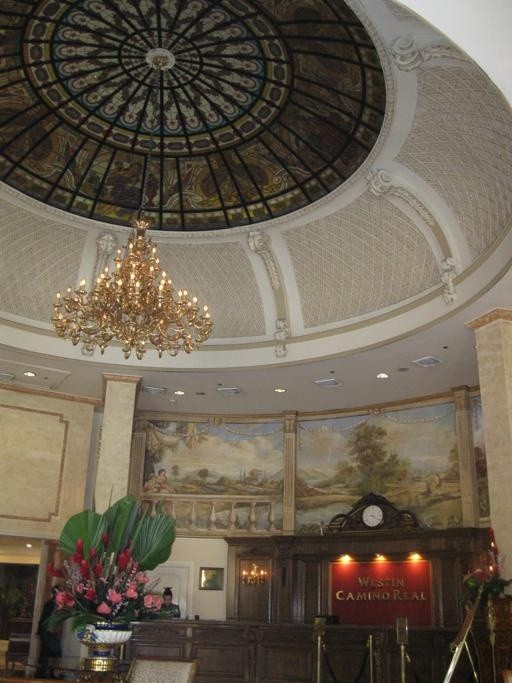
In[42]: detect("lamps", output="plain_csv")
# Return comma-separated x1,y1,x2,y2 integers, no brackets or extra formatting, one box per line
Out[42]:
49,48,213,360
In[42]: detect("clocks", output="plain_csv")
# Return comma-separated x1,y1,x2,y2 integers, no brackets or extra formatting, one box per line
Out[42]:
362,504,385,528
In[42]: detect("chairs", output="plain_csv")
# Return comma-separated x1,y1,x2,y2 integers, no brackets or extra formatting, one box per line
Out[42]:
122,653,202,683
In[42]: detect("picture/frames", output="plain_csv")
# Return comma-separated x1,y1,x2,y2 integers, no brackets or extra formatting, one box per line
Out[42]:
200,567,225,590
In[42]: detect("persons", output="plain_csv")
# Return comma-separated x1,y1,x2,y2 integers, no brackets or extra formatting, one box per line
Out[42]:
38,584,63,678
162,587,181,617
157,468,171,495
143,472,161,493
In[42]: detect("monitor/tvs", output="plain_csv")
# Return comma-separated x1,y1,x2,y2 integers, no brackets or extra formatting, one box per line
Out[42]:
316,615,338,625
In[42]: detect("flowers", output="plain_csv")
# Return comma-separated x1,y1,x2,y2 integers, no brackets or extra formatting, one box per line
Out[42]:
50,532,165,620
461,548,511,599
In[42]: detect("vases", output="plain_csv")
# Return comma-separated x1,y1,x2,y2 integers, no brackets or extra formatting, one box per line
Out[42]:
73,615,137,674
